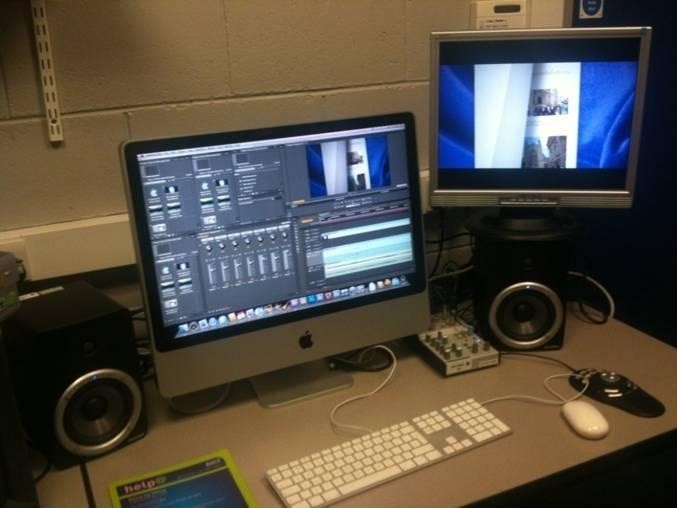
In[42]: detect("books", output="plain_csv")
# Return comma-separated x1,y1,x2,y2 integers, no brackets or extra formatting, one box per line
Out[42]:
107,446,257,507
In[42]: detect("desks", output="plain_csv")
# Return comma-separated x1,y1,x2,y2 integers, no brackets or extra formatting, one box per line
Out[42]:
32,300,677,508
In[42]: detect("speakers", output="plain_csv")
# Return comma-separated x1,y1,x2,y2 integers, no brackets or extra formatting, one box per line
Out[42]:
474,247,569,351
3,276,149,471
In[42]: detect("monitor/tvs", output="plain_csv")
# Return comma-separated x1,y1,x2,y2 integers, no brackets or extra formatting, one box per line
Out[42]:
428,25,653,241
118,109,432,409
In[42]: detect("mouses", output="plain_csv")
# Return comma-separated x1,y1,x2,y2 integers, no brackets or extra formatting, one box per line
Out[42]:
561,401,610,440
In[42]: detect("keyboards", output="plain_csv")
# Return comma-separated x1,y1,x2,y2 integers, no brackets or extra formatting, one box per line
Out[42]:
264,397,515,508
